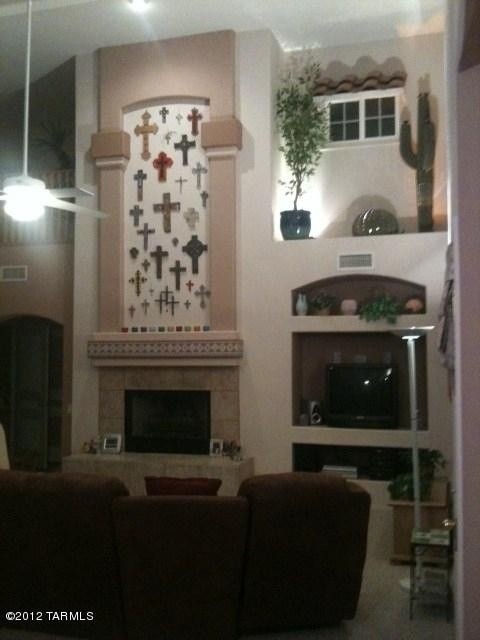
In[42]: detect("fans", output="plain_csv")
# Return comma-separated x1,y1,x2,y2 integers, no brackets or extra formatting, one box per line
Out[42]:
1,173,109,224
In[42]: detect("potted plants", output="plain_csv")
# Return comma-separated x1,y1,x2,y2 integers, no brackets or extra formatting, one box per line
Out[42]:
389,447,448,500
311,293,337,314
275,49,332,240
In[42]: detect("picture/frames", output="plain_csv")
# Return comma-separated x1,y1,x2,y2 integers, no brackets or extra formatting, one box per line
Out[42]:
209,438,224,457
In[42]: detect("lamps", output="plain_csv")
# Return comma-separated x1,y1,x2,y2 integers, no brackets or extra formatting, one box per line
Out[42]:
6,2,43,225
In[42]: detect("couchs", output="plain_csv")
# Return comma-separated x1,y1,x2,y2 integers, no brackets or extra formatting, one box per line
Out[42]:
0,467,374,639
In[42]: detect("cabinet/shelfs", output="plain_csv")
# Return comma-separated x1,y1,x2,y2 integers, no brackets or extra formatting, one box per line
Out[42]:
389,479,451,565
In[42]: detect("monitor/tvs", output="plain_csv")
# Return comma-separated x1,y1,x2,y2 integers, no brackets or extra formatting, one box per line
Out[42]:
326,360,399,428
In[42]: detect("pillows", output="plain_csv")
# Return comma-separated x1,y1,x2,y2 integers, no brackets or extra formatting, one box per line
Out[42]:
143,476,223,495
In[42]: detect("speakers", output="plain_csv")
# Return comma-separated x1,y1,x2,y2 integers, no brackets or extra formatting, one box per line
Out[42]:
309,399,323,424
